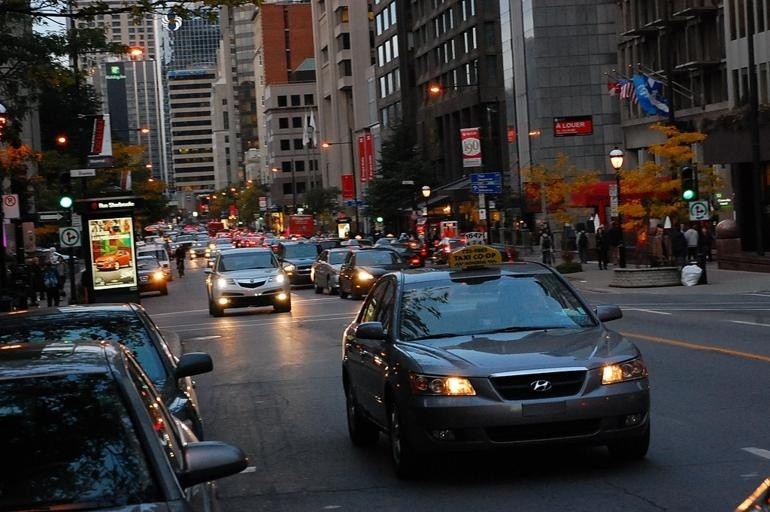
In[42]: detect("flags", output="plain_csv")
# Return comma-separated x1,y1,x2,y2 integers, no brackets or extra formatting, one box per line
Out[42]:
607,71,675,118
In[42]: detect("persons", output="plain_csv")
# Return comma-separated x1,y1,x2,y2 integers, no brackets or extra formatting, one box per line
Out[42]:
175,246,186,275
1,245,67,310
352,217,717,270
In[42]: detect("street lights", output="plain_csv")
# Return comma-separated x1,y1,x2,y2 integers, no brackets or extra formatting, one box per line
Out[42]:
324,126,360,235
429,58,494,244
271,158,297,213
128,163,153,169
66,46,145,308
108,126,152,136
205,177,254,219
608,144,629,267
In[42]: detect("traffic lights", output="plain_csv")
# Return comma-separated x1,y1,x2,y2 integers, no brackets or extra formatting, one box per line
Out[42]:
60,168,76,226
681,165,696,202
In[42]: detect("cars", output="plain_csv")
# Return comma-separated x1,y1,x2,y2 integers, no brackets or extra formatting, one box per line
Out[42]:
39,248,81,265
339,245,655,478
